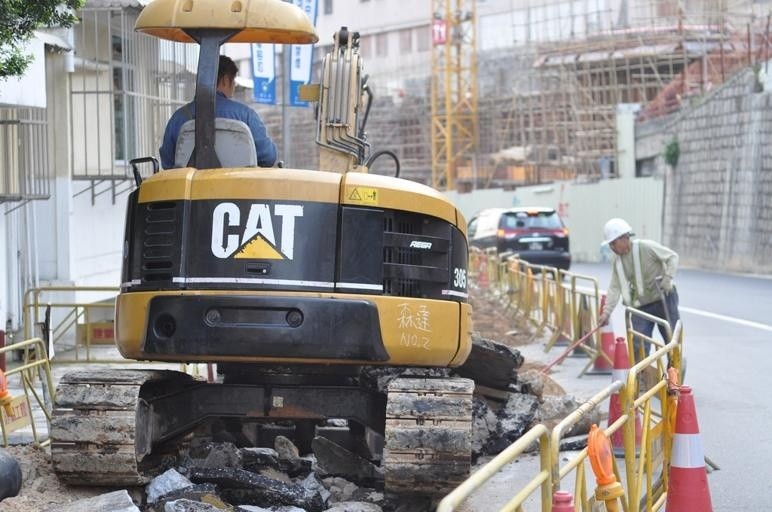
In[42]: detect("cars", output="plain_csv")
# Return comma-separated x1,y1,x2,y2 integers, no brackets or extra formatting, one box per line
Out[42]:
462,203,575,282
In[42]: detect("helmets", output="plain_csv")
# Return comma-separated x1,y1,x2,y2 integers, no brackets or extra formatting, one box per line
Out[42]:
602,219,631,243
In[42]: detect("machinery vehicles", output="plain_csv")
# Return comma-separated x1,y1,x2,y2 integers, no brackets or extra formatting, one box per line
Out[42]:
46,2,491,506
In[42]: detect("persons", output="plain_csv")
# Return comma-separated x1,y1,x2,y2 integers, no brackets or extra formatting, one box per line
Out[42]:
597,217,682,370
158,53,278,169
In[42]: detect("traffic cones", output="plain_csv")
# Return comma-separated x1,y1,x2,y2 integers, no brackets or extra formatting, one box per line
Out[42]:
477,249,491,294
606,336,647,457
664,383,713,512
590,293,617,372
